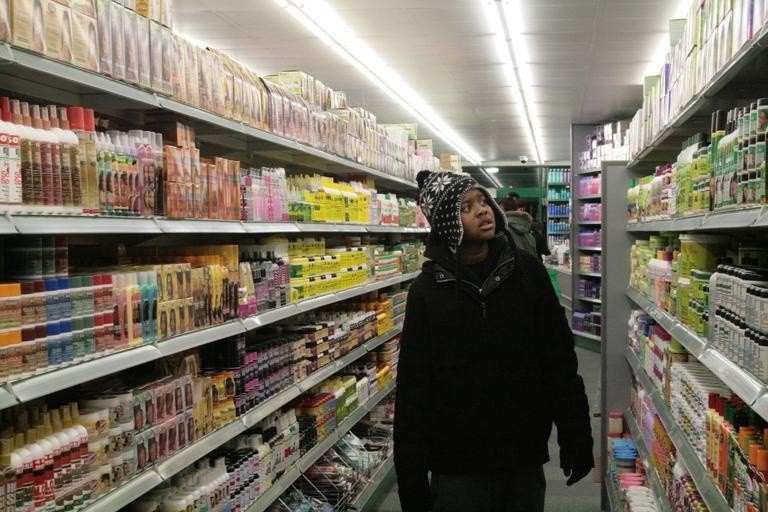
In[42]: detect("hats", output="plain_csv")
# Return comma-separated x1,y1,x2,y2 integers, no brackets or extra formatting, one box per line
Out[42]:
415,171,509,252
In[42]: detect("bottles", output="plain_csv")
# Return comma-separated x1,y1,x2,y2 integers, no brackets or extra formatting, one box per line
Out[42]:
704,392,768,512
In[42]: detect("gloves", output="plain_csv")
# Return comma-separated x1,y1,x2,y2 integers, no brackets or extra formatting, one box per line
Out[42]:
560,468,593,487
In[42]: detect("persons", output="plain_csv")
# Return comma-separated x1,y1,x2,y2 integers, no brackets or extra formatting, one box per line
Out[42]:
393,170,595,512
494,197,538,262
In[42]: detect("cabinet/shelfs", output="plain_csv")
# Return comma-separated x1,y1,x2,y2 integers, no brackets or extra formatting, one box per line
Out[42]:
571,126,626,352
603,1,762,512
0,0,468,510
543,168,572,253
